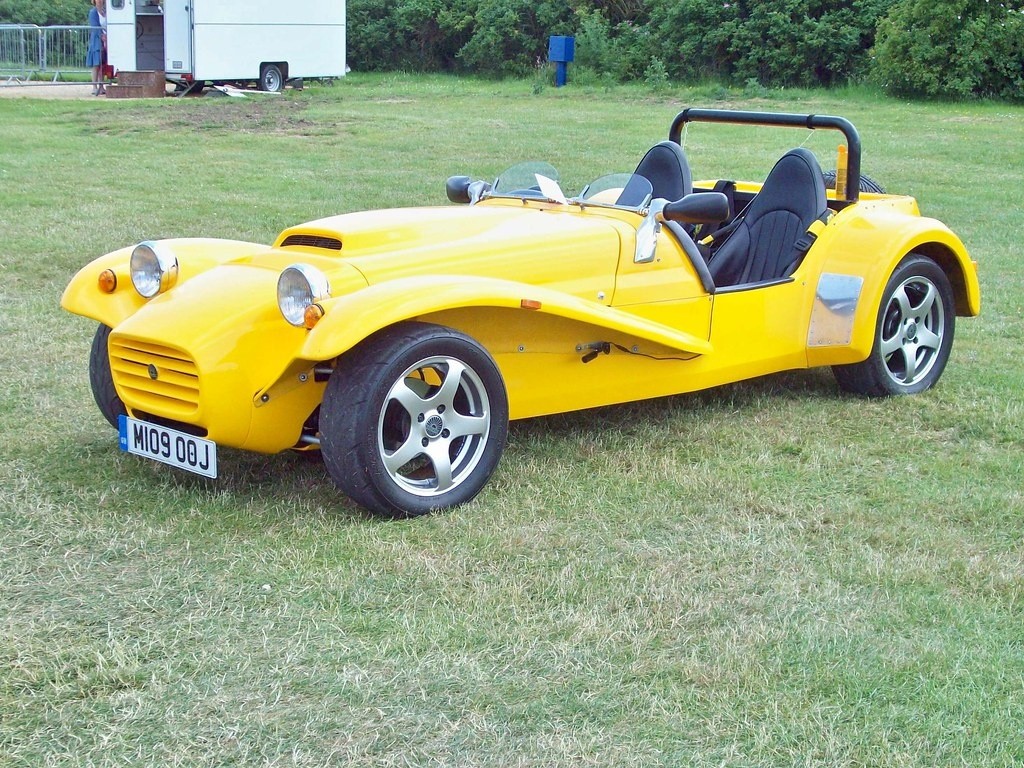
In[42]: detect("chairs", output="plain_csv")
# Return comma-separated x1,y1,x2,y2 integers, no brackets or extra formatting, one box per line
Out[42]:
706,147,827,287
615,141,694,232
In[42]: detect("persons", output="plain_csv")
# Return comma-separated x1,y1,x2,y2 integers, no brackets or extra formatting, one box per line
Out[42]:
85,0,107,95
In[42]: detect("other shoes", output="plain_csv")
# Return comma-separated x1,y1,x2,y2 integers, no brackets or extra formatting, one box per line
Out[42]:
92,88,106,94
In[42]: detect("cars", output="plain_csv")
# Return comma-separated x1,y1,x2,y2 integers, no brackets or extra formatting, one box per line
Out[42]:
55,104,982,518
